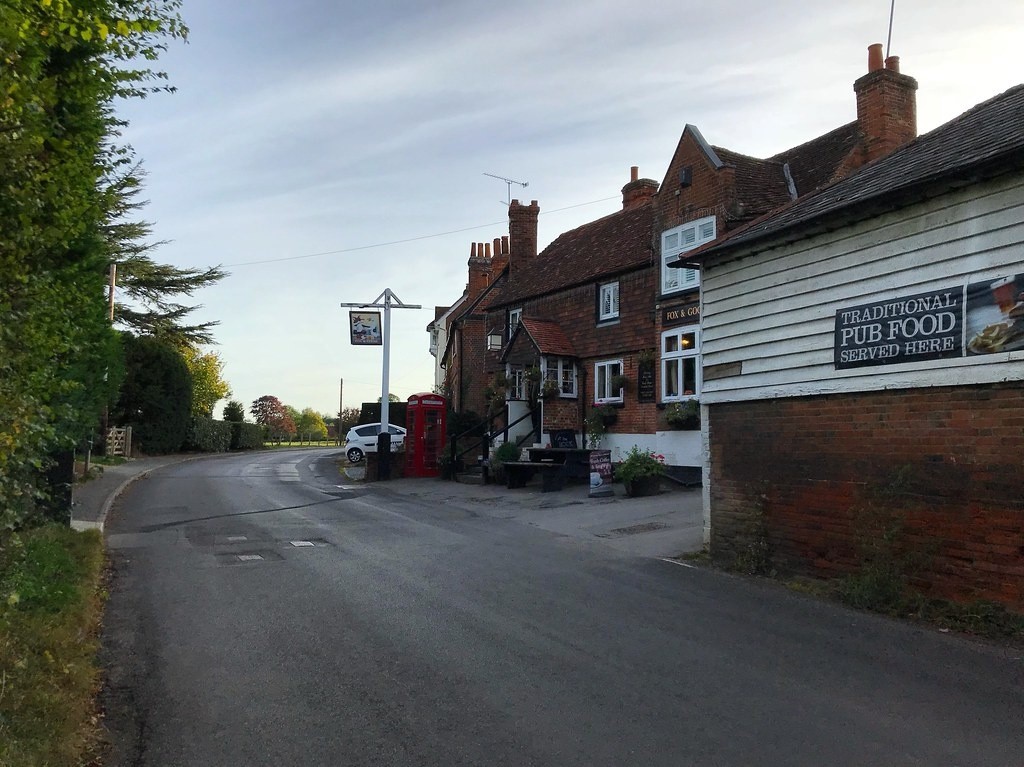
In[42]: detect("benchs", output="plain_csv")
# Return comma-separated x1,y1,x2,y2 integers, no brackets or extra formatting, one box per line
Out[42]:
503,461,578,492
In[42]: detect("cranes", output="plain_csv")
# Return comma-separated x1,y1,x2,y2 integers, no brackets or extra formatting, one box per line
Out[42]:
482,173,529,206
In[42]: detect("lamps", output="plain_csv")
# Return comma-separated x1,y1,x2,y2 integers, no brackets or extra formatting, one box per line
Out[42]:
486,322,521,352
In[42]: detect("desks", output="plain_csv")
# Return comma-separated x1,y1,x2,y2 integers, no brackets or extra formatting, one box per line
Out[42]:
527,448,612,482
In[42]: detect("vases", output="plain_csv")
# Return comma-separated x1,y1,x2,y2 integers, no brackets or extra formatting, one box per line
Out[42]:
613,475,668,497
674,416,700,430
603,414,617,425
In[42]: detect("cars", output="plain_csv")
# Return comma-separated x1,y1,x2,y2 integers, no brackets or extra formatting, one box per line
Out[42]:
344,422,410,463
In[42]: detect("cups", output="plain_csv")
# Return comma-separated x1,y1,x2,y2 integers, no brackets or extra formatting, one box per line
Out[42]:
986,275,1017,314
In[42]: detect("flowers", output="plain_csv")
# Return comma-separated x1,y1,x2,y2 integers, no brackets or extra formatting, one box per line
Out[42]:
592,399,616,416
612,442,670,489
663,398,699,426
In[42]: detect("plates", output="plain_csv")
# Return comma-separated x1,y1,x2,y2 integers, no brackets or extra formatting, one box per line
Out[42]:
967,323,1024,354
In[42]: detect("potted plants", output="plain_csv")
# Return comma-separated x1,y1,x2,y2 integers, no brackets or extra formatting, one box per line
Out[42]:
611,375,625,388
598,480,599,483
523,366,542,388
